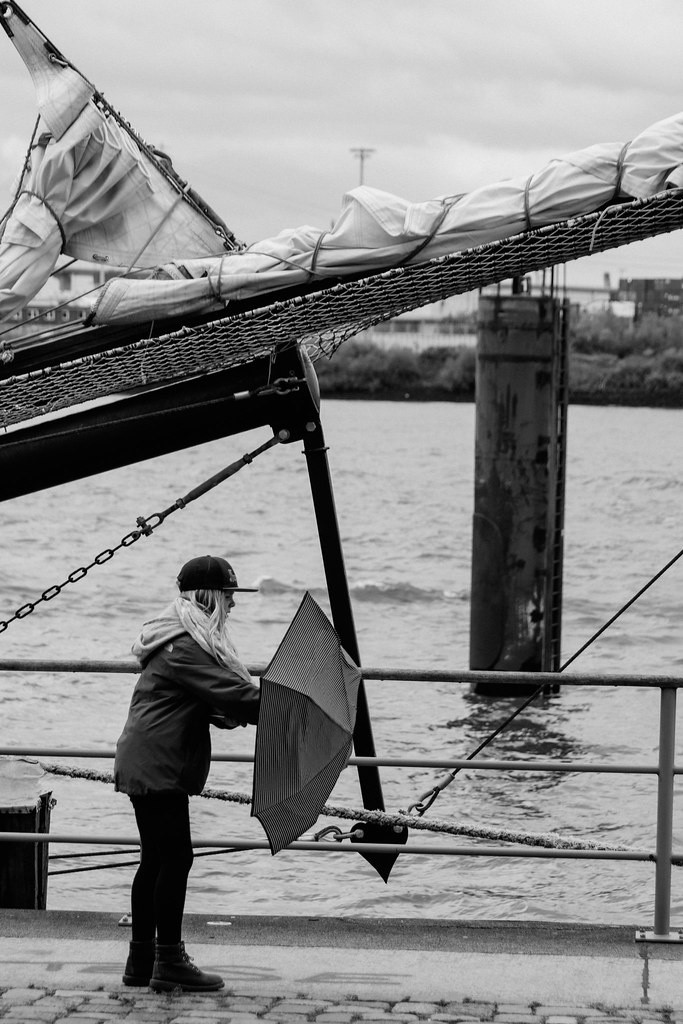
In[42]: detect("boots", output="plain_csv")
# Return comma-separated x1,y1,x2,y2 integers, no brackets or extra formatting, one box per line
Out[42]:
123,940,156,987
150,941,224,991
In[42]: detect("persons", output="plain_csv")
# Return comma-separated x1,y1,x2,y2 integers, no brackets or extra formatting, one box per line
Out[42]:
114,555,259,992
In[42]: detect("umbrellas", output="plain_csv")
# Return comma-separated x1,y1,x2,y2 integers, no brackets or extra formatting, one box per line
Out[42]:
250,590,360,856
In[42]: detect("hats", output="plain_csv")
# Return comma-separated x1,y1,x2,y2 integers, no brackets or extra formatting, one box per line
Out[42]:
177,555,259,592
